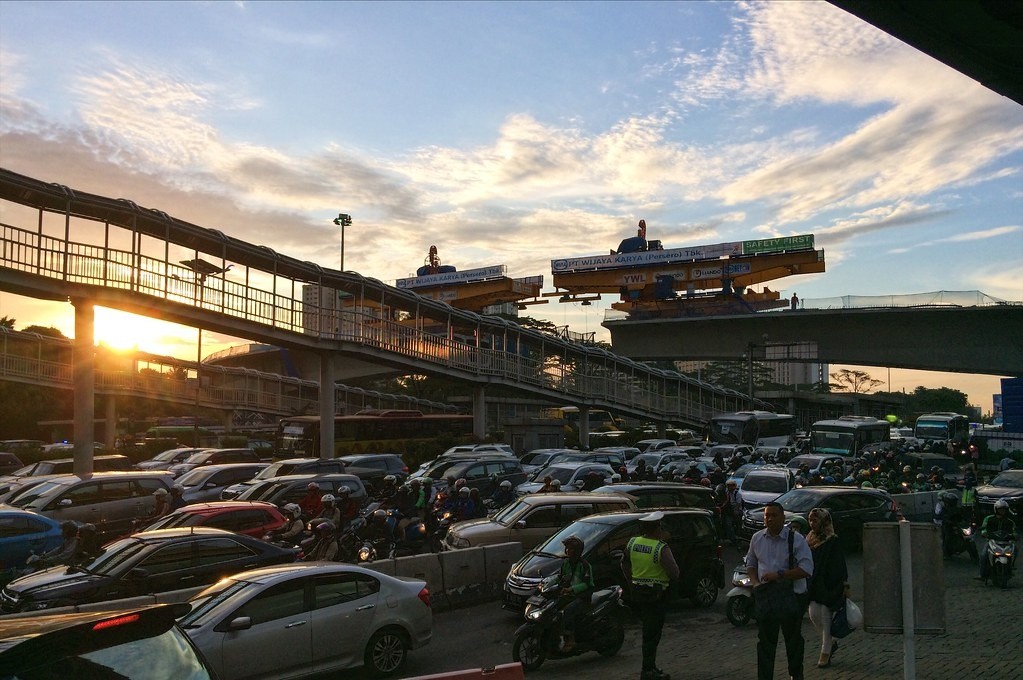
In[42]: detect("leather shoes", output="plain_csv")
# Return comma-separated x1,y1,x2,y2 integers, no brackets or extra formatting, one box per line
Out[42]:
640,666,671,680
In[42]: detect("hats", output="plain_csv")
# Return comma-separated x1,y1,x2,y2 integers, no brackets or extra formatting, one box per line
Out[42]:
85,523,96,532
639,511,665,527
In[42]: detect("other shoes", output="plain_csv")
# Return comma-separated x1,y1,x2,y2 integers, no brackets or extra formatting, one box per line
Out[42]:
562,636,575,653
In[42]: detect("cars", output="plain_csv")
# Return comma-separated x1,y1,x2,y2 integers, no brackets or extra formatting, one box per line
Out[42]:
0,441,269,555
174,563,433,680
890,428,914,443
222,443,527,512
444,429,754,552
503,507,727,617
975,468,1023,514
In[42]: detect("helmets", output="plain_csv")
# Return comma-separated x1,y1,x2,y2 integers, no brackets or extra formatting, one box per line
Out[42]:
171,484,185,495
284,503,302,519
383,475,396,485
321,494,336,507
993,500,1010,515
937,489,959,503
550,479,561,490
338,485,351,498
589,438,973,494
316,523,336,538
786,516,808,534
488,472,498,483
152,487,167,501
398,475,466,494
500,480,511,492
308,482,319,494
459,487,470,498
562,534,584,557
58,520,78,535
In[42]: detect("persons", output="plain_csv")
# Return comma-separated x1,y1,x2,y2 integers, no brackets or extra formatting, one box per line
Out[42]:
41,521,98,567
789,516,810,539
170,483,188,510
746,502,814,680
141,488,169,525
934,491,961,559
793,437,979,496
272,470,518,560
536,448,792,546
961,480,979,510
977,500,1018,582
805,508,849,667
539,536,594,656
625,510,678,679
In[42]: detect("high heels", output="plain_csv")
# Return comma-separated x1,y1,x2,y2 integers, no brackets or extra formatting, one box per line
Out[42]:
817,640,838,668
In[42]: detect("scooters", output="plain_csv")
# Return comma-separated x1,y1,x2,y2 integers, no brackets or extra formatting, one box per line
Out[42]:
725,559,760,627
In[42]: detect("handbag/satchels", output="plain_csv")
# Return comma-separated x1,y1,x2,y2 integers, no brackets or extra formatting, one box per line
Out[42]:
786,592,811,618
830,597,863,638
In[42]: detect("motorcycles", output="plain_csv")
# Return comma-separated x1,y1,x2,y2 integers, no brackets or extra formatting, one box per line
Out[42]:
264,496,501,564
795,445,984,497
982,530,1017,587
511,570,625,671
945,521,980,562
14,550,91,580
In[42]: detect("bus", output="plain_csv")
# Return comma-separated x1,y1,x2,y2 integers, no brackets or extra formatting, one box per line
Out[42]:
914,413,969,446
271,409,474,463
703,410,797,447
810,415,890,458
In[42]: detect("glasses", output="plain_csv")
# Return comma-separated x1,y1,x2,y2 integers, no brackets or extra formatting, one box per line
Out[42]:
156,495,159,496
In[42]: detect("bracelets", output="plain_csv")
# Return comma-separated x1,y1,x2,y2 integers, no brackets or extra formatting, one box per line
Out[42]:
777,569,784,578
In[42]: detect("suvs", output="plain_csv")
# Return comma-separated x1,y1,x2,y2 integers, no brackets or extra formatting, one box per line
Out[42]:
0,601,216,680
740,486,906,552
